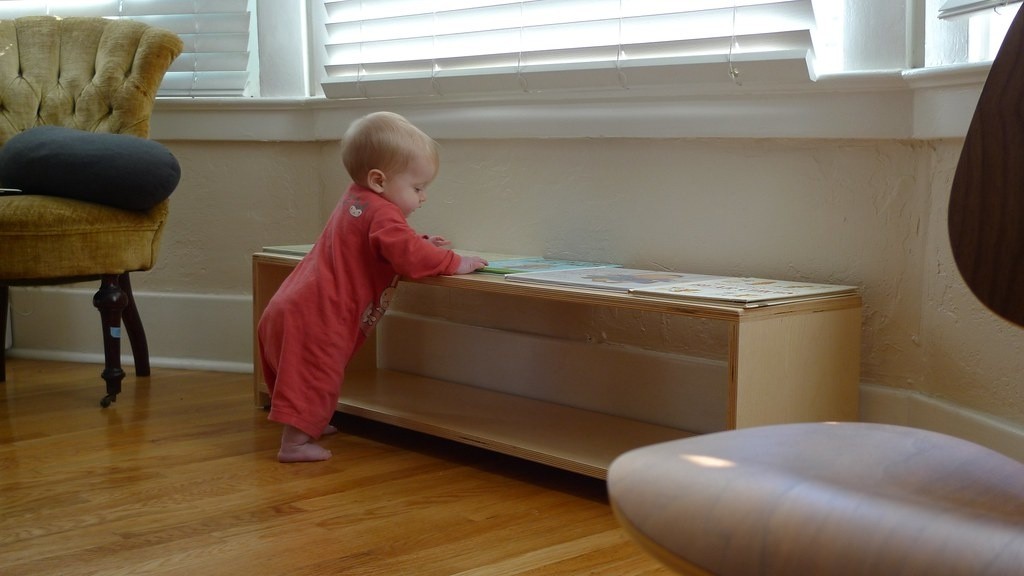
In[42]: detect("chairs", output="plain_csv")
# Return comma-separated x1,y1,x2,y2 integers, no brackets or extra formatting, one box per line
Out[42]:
605,0,1024,576
0,13,184,409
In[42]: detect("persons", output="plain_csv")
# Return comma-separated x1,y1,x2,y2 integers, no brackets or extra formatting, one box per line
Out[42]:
258,111,489,461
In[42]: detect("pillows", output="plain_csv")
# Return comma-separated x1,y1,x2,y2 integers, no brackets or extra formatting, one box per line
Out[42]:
0,124,181,212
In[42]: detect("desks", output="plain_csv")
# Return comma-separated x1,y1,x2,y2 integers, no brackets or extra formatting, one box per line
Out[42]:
249,248,864,485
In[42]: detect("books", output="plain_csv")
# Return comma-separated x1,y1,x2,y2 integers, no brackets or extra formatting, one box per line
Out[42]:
627,276,859,308
474,258,623,276
263,244,316,256
505,267,728,294
452,249,542,260
